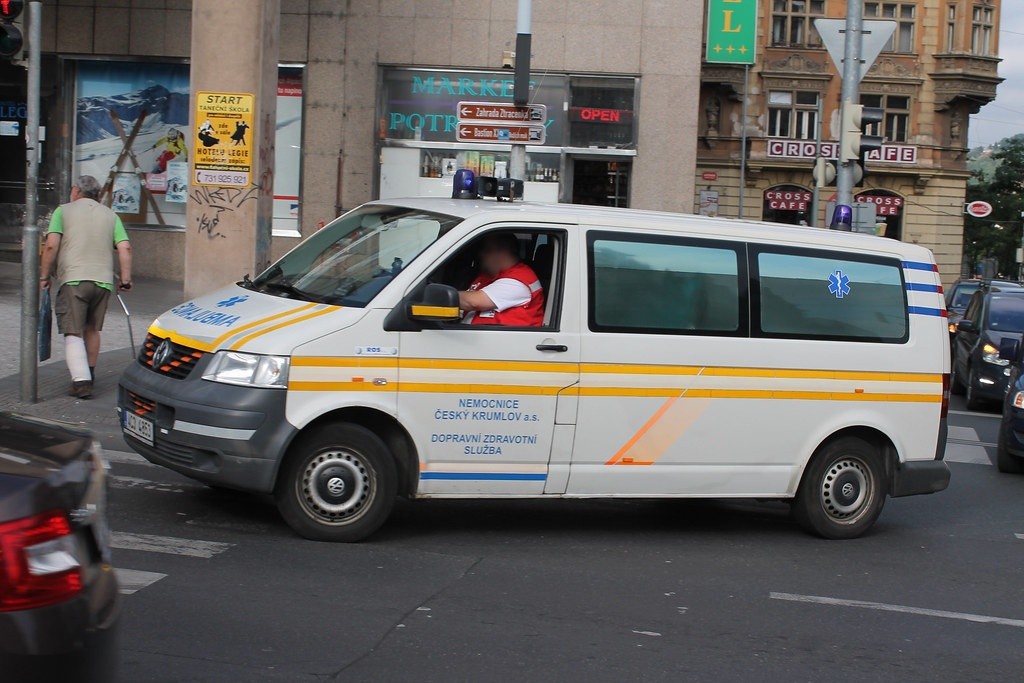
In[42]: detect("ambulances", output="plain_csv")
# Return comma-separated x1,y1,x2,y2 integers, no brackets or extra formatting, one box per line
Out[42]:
116,167,953,540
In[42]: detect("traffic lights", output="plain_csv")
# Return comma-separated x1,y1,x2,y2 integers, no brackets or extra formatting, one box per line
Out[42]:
0,1,25,61
846,105,884,160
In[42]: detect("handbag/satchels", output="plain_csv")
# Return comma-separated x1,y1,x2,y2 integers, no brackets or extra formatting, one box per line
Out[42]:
39,287,52,363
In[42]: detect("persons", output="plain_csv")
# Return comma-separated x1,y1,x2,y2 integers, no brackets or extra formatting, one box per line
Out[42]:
458,232,544,327
152,128,188,173
39,174,133,398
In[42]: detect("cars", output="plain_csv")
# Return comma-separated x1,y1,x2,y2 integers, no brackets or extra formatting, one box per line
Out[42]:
999,338,1024,472
946,278,1021,337
956,285,1024,411
0,411,118,682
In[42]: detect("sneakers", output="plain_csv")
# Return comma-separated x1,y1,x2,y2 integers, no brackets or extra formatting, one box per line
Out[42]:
67,380,93,397
